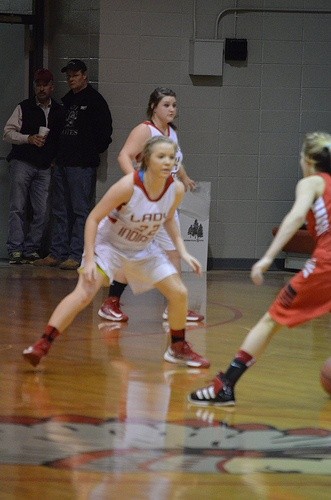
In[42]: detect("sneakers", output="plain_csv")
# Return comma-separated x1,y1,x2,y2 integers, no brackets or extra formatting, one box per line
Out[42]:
22,337,52,368
188,373,237,408
97,297,129,322
163,341,210,369
162,303,206,323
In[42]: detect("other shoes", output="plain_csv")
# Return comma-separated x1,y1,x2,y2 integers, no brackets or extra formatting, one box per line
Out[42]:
34,257,60,265
23,253,40,263
60,259,81,269
9,252,22,264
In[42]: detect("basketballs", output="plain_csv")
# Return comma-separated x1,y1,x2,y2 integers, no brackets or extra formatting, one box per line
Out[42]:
319,356,331,395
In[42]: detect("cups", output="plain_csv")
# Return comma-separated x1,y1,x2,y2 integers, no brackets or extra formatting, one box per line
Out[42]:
37,127,50,146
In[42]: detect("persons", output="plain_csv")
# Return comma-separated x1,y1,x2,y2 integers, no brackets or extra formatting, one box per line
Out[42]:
188,131,331,406
21,135,211,369
3,68,60,263
34,60,113,270
98,87,204,322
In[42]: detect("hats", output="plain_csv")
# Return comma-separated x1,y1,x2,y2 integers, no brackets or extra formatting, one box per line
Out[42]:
61,60,87,73
33,68,54,82
